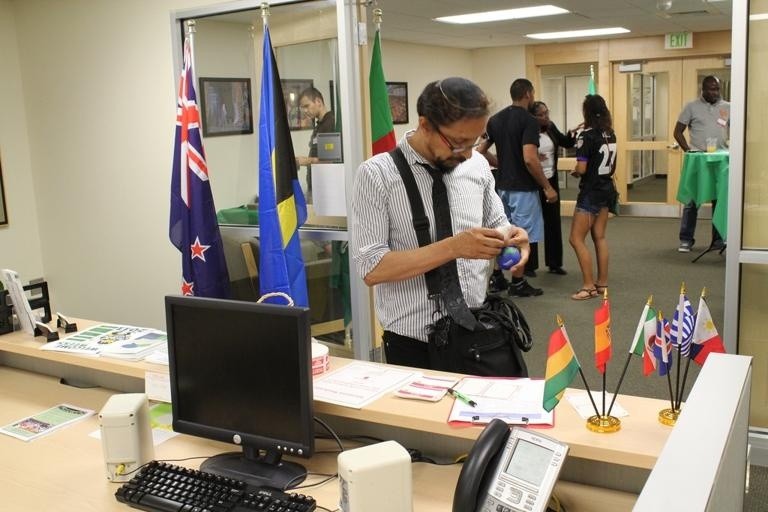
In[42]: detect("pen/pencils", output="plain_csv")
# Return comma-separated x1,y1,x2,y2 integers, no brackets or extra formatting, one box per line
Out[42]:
446,387,477,408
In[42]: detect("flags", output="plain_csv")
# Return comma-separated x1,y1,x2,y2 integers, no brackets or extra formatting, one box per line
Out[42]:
593,301,613,372
541,324,580,413
368,31,396,157
589,78,595,96
257,24,309,310
167,35,231,300
628,293,726,375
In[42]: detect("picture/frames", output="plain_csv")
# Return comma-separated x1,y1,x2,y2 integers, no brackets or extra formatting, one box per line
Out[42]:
279,78,316,132
383,80,409,125
197,76,255,139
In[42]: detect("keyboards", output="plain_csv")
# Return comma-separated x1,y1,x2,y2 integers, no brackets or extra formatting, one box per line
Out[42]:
114,460,316,512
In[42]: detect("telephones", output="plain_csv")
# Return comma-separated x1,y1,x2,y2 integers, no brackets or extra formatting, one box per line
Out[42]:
452,419,570,512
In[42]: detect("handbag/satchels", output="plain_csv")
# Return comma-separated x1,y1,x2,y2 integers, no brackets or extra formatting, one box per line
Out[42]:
427,295,527,376
608,192,619,217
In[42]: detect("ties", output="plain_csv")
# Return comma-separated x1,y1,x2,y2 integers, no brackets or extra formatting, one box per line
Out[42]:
426,168,477,332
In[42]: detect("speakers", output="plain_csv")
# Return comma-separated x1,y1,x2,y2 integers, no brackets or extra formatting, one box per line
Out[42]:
337,439,414,512
98,393,155,483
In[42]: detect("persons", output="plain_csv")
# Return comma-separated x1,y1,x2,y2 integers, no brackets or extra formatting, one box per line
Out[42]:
567,94,618,300
525,101,575,277
293,87,334,205
351,78,532,379
673,74,731,252
475,78,557,299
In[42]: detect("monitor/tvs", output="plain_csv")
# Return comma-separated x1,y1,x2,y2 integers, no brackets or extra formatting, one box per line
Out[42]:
164,295,314,491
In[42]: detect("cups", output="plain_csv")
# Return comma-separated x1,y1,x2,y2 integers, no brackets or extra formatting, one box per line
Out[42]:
706,138,716,152
312,344,330,378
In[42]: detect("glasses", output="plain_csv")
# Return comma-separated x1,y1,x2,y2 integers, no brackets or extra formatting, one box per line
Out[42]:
427,115,489,152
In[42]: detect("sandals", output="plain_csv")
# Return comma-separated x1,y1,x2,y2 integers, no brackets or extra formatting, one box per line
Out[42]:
571,285,607,300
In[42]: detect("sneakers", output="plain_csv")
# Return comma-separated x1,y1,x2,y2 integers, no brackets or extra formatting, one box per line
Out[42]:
488,274,542,298
678,242,690,252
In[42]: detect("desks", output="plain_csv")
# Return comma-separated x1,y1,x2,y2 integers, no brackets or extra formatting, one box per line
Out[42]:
674,147,729,264
0,315,691,512
